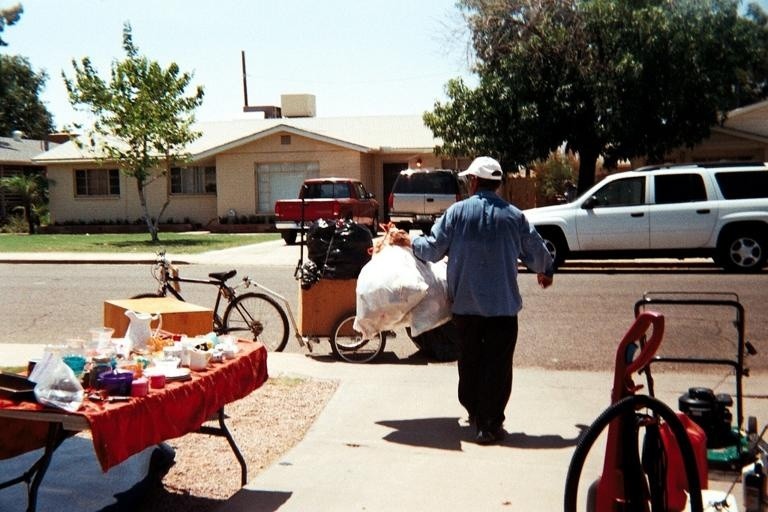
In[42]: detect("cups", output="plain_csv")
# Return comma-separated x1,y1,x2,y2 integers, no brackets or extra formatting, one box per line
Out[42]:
131,333,239,398
62,356,85,377
95,371,132,401
87,327,115,359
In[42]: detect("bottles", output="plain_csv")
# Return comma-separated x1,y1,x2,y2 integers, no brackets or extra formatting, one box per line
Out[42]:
743,464,765,512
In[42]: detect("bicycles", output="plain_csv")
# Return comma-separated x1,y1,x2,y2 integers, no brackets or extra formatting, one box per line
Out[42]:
125,251,288,353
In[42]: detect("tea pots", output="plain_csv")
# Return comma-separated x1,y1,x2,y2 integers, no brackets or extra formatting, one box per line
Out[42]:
122,309,162,350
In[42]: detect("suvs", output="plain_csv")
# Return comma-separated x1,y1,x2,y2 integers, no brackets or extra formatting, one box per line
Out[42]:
516,164,768,273
390,168,463,235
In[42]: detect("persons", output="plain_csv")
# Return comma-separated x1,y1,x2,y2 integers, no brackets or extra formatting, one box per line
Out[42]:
386,154,554,447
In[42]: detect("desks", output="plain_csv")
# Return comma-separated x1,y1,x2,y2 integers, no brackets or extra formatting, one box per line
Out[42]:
1,334,267,509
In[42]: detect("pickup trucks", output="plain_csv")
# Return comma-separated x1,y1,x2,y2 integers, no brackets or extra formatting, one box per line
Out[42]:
273,178,378,244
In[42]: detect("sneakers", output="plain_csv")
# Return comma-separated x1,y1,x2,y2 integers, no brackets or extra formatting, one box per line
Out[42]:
475,427,509,443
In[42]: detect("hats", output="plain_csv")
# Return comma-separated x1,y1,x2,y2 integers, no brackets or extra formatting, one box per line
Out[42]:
457,156,502,181
